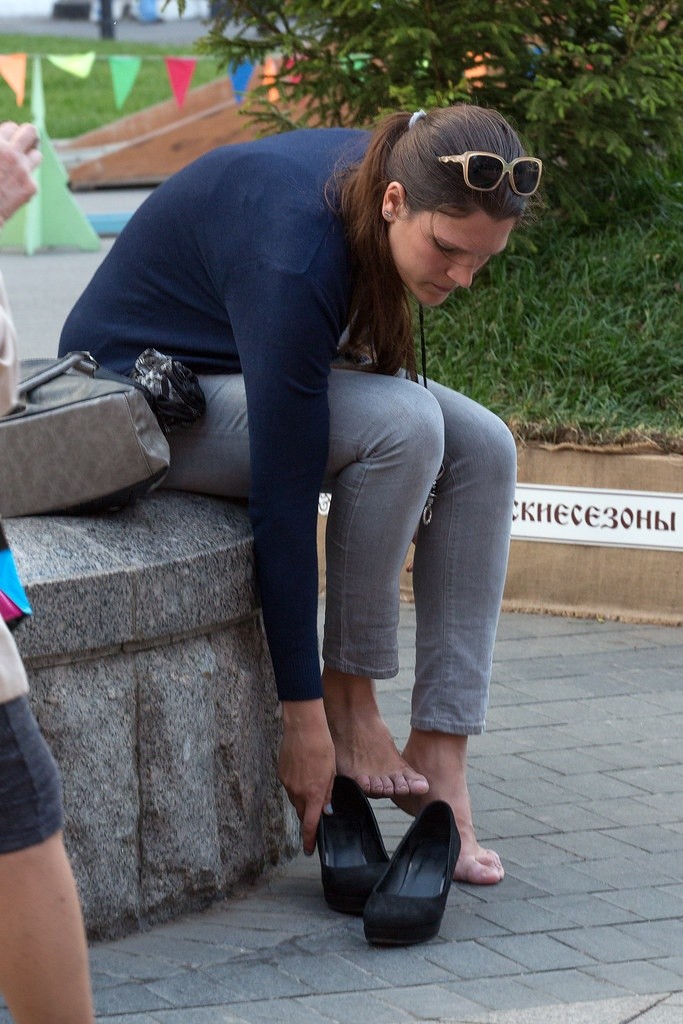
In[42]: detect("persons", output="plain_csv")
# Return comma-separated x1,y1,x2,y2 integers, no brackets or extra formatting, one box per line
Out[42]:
58,101,541,885
0,120,96,1023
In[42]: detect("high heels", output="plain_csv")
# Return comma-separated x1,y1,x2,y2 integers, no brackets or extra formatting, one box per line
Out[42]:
362,800,460,945
316,775,391,913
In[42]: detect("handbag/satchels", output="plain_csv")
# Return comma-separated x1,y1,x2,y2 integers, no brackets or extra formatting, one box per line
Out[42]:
0,346,172,519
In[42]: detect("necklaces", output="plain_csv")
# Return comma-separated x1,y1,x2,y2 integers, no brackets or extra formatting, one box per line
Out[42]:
372,298,444,525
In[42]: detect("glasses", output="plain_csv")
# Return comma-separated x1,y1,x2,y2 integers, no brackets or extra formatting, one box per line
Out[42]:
438,151,542,196
